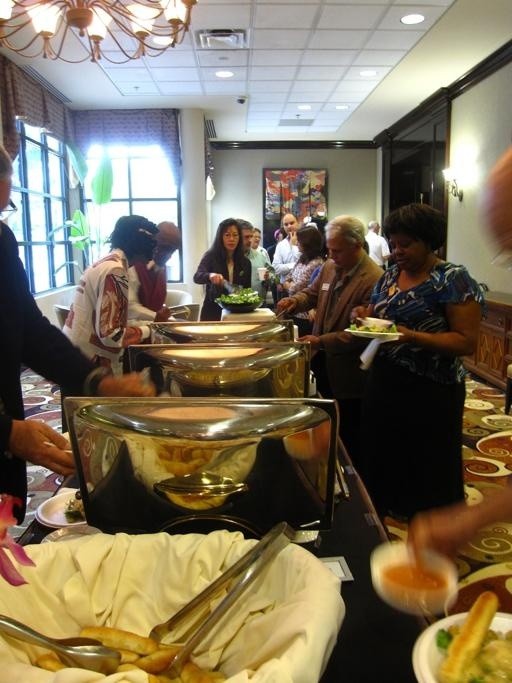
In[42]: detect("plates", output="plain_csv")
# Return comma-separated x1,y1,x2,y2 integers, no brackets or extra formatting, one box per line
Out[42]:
343,328,404,339
356,315,392,326
35,491,90,528
40,525,104,545
410,610,512,682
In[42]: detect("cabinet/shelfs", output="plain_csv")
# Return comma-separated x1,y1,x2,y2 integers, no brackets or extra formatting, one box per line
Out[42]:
459,285,512,395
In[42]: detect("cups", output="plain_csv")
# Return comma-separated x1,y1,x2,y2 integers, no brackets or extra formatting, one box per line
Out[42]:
258,267,267,280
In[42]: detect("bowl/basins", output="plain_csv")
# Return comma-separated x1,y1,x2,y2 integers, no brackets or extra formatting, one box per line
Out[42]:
368,541,459,612
216,299,261,311
152,514,264,541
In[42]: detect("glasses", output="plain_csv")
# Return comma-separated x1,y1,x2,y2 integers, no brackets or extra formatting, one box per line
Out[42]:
0,198,17,221
223,232,240,239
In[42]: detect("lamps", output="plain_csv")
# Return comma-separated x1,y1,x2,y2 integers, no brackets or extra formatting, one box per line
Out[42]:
440,161,463,203
1,0,199,64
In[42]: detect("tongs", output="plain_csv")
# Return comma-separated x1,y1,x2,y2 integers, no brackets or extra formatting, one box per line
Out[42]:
1,616,121,673
146,520,295,678
223,279,243,292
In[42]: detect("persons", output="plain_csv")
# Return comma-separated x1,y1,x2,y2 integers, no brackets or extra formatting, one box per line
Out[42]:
275,215,385,452
351,203,484,521
64,215,180,375
266,213,391,338
193,218,271,321
410,150,511,567
0,147,152,523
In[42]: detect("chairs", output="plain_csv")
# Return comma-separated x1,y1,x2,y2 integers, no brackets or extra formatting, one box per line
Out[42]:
162,289,194,309
167,302,200,322
51,301,71,331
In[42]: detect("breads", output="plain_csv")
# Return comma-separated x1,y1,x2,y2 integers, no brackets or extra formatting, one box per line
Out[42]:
435,591,500,681
32,624,225,683
156,444,215,477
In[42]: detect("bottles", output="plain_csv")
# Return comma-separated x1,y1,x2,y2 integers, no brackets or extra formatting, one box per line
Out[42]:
267,275,276,304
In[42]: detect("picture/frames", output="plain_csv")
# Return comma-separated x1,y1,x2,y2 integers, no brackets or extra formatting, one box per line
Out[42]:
260,166,329,261
